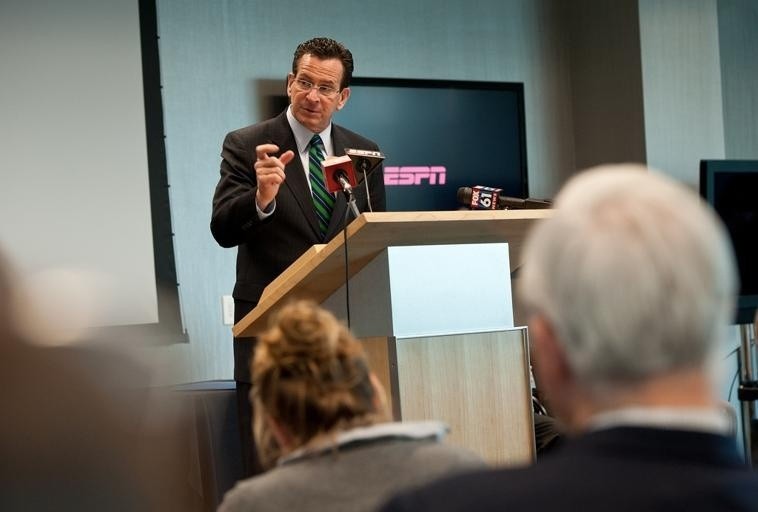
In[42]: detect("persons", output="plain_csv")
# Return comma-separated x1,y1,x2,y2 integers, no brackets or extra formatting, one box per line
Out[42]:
219,301,485,512
210,37,386,469
382,159,758,512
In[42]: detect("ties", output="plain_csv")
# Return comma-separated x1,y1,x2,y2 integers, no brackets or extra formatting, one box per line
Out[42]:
307,135,337,235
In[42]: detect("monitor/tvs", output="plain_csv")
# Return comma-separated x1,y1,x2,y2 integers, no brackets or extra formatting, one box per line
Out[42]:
332,77,529,211
699,156,758,324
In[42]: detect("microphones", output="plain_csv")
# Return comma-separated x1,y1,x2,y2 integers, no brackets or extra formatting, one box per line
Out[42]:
344,146,386,211
327,156,353,193
455,185,551,211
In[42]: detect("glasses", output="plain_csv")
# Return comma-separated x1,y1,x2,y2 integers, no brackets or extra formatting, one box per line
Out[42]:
292,77,341,97
